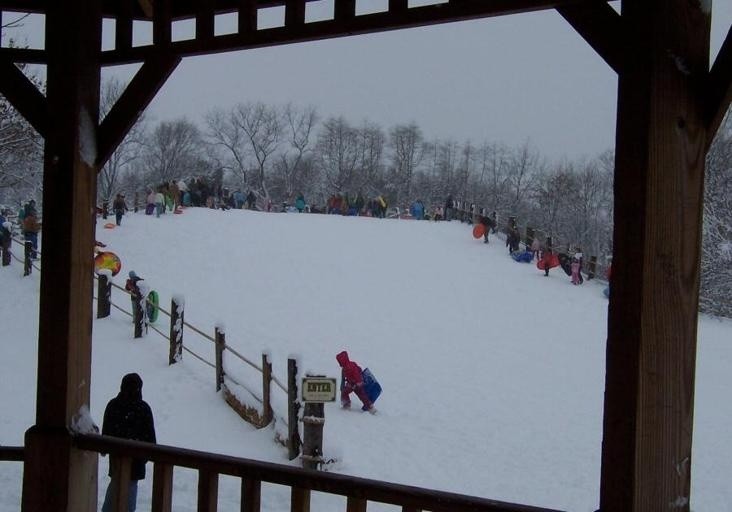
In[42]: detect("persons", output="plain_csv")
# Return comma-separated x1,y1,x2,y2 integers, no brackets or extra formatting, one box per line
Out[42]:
324,193,388,218
414,199,423,220
296,192,305,212
444,195,454,220
126,271,145,301
113,194,128,225
146,180,256,217
24,200,41,261
100,373,157,511
337,351,376,412
602,254,612,297
477,214,583,285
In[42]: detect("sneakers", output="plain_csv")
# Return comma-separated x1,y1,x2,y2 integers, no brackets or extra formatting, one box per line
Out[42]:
340,407,351,411
369,407,377,414
